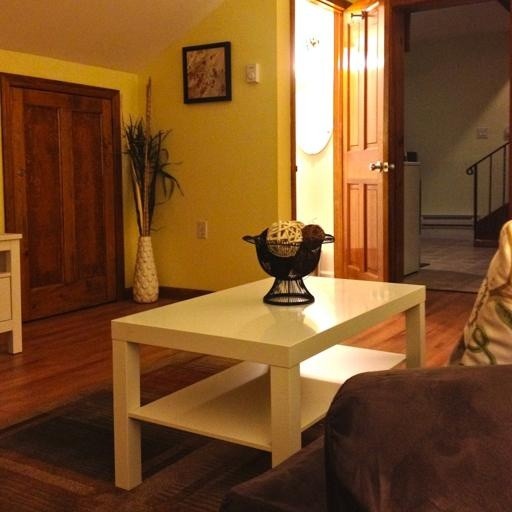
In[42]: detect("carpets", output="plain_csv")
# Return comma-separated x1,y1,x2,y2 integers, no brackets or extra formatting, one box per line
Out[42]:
1,352,236,488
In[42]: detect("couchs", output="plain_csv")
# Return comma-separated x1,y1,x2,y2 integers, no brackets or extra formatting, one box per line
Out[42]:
223,339,511,510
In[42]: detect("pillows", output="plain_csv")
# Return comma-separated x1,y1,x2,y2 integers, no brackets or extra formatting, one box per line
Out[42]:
444,218,512,368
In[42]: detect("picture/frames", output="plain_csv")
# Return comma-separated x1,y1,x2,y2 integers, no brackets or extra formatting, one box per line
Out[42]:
181,42,232,104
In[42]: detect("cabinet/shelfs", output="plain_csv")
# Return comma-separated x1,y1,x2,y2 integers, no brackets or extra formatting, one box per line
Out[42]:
402,160,424,278
2,73,123,323
0,233,23,354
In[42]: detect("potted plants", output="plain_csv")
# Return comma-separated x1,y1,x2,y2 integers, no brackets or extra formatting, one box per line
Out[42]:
121,77,183,303
